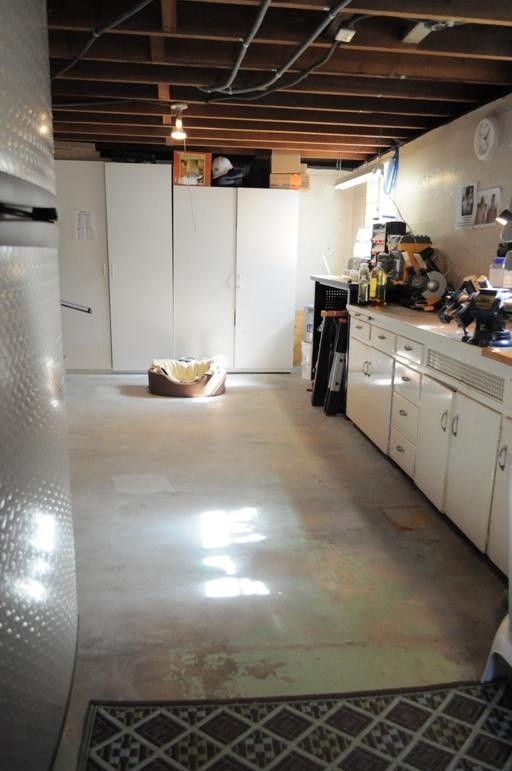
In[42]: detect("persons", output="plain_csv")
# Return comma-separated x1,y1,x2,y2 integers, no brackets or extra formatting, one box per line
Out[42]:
181,160,205,185
475,193,497,225
462,187,471,215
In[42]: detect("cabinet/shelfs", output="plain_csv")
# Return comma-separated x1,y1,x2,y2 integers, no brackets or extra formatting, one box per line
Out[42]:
52,162,173,373
486,416,511,580
345,316,424,481
173,183,300,374
413,375,502,556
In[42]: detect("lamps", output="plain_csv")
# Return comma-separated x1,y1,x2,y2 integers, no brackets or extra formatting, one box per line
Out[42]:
169,103,188,140
332,126,385,190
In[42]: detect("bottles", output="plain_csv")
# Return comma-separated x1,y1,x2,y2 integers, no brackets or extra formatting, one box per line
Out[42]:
357,262,386,304
489,257,507,289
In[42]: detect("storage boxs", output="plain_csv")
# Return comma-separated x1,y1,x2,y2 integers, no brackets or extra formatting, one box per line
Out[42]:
269,154,311,190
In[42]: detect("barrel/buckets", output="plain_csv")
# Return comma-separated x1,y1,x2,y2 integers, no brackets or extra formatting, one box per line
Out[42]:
300,341,314,380
303,304,315,343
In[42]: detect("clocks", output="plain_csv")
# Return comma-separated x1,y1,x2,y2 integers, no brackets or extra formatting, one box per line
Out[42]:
472,117,500,160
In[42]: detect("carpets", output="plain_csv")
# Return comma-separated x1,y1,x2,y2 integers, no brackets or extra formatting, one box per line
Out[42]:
74,679,511,771
